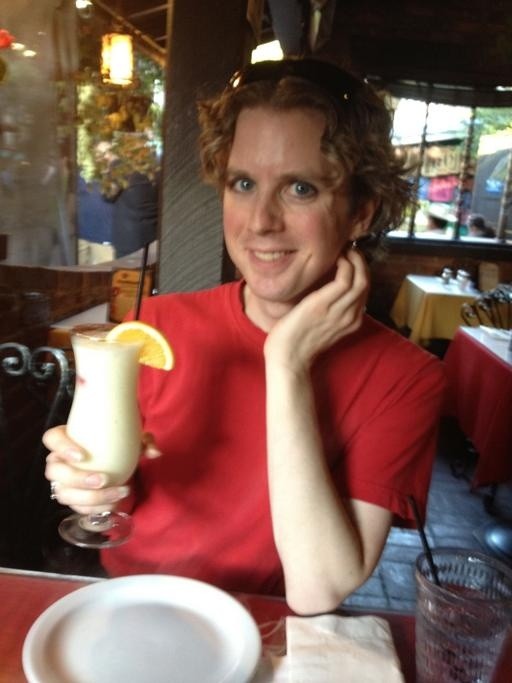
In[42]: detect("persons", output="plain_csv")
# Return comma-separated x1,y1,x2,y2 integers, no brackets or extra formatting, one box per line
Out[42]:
421,203,458,237
42,58,448,616
466,214,496,237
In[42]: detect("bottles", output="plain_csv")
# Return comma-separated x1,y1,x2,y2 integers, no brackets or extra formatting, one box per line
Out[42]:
441,268,470,290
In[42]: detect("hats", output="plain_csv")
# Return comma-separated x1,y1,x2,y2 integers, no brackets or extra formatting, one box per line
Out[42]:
424,203,458,222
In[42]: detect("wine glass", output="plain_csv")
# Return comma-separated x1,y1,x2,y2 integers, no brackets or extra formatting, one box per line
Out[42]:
56,325,143,549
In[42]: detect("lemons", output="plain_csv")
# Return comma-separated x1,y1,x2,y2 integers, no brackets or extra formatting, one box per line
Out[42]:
105,319,175,372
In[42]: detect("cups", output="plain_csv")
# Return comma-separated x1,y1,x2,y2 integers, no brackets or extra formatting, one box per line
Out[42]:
415,547,511,683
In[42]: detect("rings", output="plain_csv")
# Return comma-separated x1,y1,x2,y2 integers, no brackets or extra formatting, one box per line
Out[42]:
50,482,56,500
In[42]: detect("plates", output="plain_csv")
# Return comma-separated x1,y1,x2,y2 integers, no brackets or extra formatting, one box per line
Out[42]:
21,574,263,683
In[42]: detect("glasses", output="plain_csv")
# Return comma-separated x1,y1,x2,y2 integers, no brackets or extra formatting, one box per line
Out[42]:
217,59,374,127
